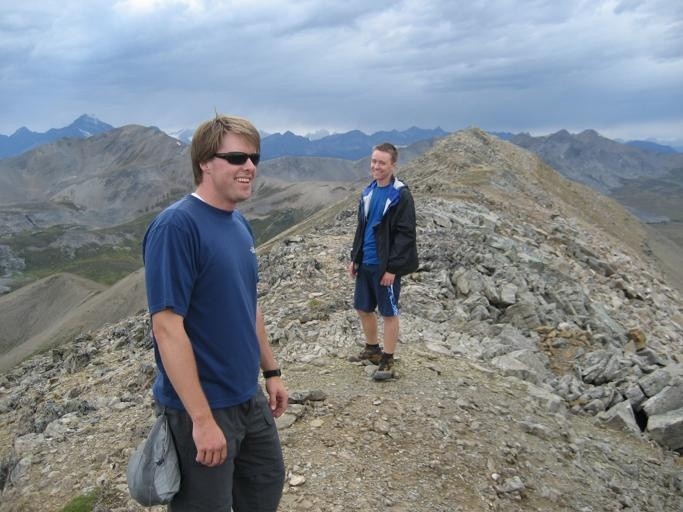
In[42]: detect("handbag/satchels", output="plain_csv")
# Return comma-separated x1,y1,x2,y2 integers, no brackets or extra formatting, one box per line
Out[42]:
127,414,180,507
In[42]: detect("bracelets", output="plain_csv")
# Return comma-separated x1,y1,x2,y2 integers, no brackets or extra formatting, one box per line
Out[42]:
262,369,281,378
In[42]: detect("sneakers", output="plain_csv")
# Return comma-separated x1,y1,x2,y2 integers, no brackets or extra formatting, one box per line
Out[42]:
349,346,381,362
373,357,395,379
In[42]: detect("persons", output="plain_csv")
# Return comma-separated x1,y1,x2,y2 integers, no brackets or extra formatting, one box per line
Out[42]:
347,143,420,380
141,109,290,512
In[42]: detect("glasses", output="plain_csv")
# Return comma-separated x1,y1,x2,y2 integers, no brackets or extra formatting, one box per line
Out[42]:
209,151,260,165
